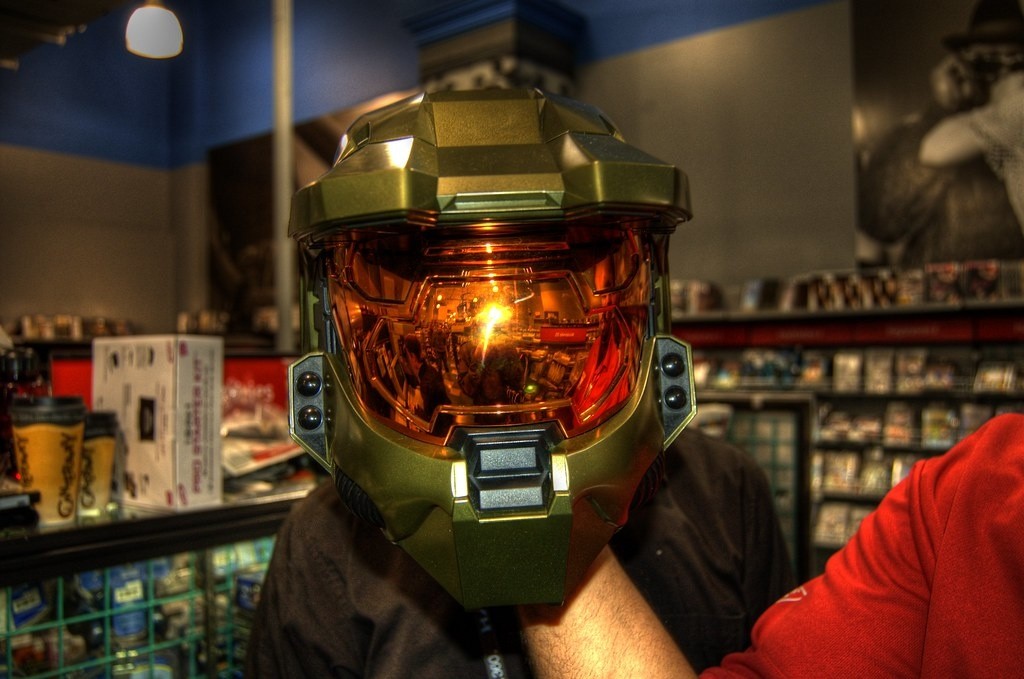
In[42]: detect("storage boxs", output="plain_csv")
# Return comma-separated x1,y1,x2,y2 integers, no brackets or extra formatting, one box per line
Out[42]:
93,335,221,511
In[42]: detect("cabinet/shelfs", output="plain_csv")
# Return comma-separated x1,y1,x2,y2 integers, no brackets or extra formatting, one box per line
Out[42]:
0,487,308,679
666,317,1023,553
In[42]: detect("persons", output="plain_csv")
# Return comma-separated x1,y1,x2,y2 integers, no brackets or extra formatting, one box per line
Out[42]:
514,413,1023,678
241,84,796,678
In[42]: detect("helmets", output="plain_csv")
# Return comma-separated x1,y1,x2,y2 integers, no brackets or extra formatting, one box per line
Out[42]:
287,82,693,613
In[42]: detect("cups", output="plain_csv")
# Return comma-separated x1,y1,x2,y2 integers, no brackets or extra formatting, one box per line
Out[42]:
8,396,84,526
75,412,118,517
